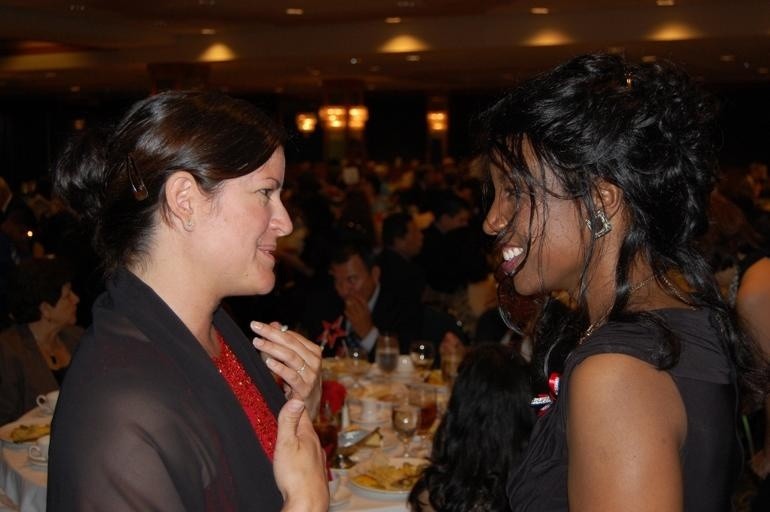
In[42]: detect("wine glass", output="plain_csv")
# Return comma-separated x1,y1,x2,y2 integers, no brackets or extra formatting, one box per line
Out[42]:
347,337,439,459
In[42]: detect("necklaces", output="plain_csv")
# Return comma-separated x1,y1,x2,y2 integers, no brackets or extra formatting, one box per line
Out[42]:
579,272,665,344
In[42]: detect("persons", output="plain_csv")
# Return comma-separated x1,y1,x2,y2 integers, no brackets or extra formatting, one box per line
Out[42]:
228,150,589,369
466,48,770,509
1,176,108,420
406,340,554,512
704,162,770,420
45,88,333,511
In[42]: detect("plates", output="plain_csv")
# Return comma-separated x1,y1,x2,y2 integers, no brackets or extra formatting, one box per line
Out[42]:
348,456,432,501
328,486,350,507
28,460,48,466
337,427,380,468
0,417,52,448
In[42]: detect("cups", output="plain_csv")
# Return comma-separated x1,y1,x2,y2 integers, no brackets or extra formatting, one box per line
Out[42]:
36,389,60,411
27,435,50,462
328,472,341,500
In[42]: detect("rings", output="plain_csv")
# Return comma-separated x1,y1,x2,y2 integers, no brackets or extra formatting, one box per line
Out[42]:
296,361,307,373
